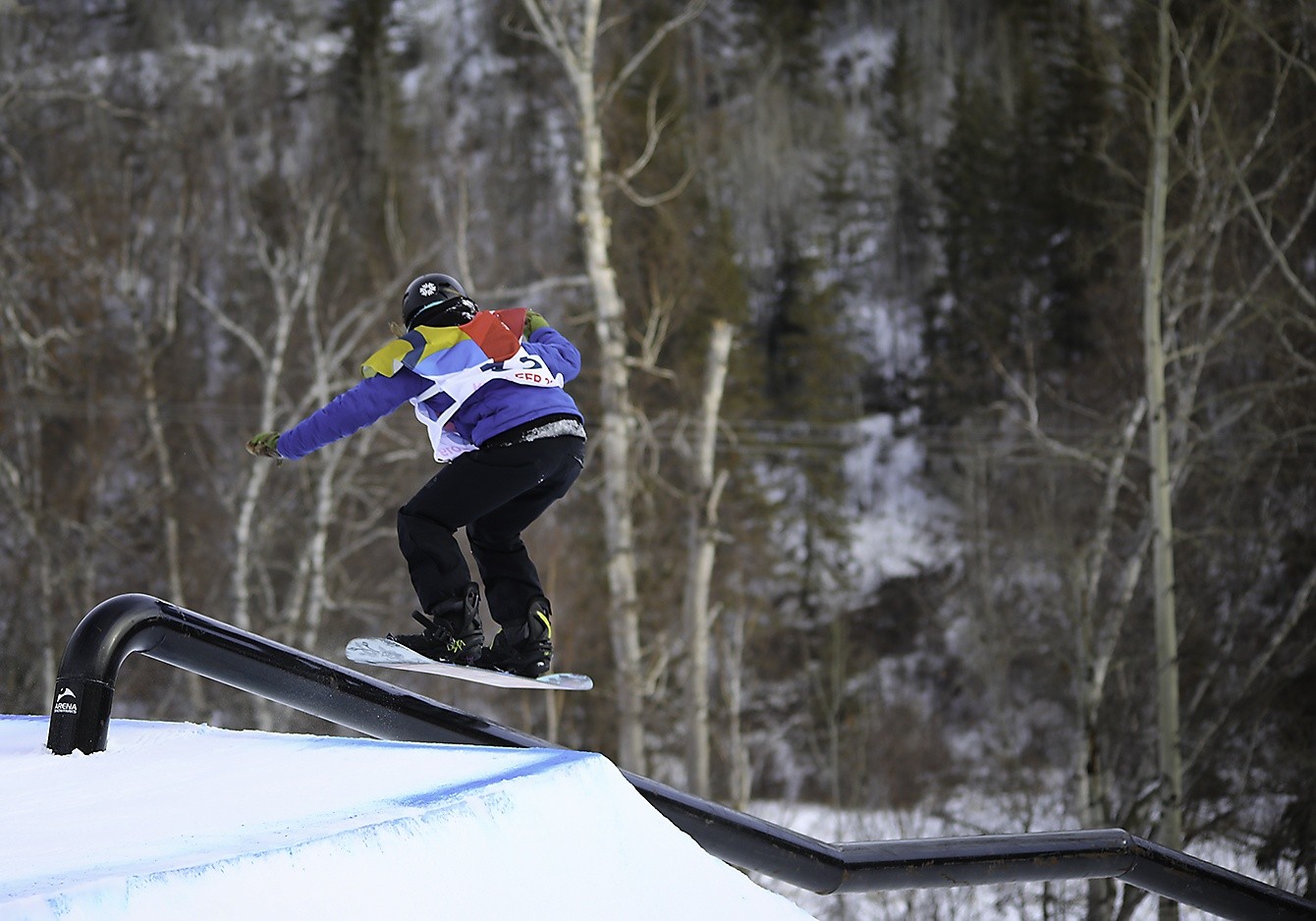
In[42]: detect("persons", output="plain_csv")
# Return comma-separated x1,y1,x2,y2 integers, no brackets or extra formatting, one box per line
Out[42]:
244,273,588,676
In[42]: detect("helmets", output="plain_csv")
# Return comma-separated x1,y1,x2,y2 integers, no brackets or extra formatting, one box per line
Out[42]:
402,273,467,330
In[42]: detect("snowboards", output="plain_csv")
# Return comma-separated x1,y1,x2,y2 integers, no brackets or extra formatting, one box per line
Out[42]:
345,636,595,691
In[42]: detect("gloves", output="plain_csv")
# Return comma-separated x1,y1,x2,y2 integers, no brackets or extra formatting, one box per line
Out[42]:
246,432,285,468
523,310,549,339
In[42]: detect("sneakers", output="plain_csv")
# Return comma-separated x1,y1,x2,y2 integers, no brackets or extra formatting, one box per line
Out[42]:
483,597,553,676
394,583,484,663
